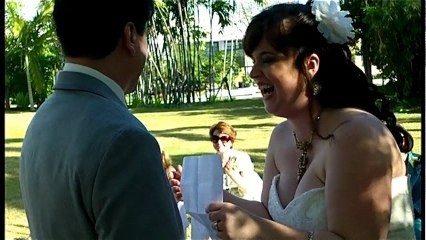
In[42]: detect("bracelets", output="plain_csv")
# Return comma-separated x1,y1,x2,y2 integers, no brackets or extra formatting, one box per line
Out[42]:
304,231,314,240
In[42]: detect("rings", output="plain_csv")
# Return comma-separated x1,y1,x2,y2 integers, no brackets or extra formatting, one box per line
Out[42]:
213,222,218,231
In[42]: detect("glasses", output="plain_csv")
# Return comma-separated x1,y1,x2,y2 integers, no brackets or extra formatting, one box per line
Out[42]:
211,135,229,142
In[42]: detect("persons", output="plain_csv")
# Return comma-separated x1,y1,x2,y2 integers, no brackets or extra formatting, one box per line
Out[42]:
208,121,263,203
169,0,418,240
18,0,187,240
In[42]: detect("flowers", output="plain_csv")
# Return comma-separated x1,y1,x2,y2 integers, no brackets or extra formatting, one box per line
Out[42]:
410,155,422,175
310,0,355,44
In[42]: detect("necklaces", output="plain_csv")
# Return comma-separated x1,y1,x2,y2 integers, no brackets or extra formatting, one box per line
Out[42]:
292,112,321,184
